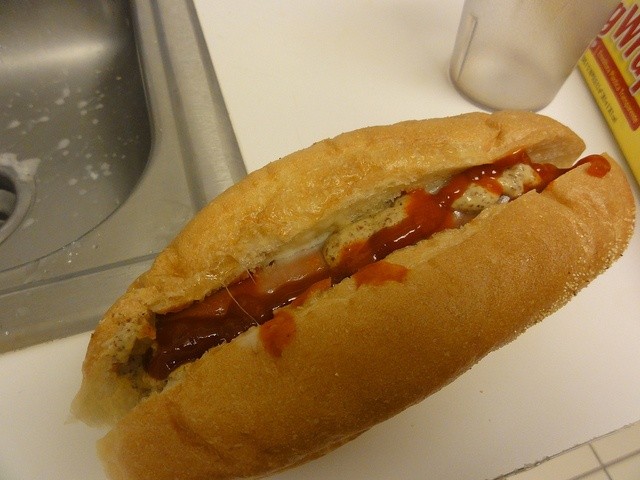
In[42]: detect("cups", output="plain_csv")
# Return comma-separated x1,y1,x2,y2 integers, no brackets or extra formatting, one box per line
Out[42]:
450,1,621,112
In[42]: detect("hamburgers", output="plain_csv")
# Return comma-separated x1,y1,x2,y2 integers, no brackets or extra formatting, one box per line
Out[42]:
68,110,636,477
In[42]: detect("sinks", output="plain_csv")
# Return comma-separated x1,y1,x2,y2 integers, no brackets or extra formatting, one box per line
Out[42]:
1,0,248,354
